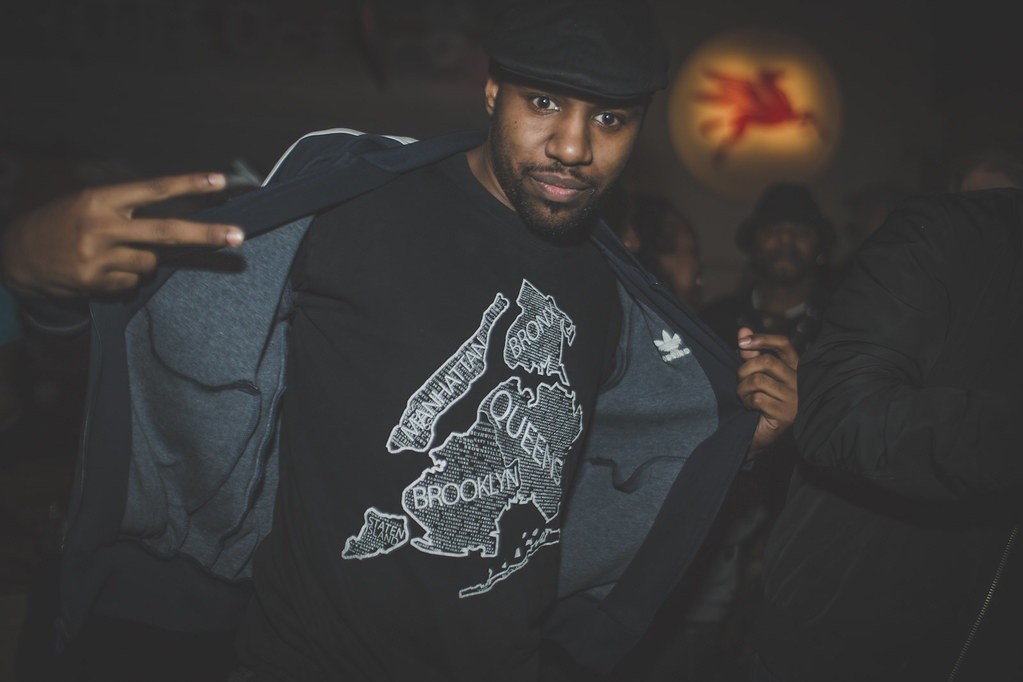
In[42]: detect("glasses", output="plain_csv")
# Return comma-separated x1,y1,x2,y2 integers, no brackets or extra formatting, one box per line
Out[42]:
757,223,816,243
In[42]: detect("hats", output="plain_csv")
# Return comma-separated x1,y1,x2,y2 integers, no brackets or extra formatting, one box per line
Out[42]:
733,184,836,256
484,0,672,100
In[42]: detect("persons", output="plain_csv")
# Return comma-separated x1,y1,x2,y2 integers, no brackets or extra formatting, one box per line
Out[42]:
761,163,1023,681
599,178,834,682
1,0,798,682
